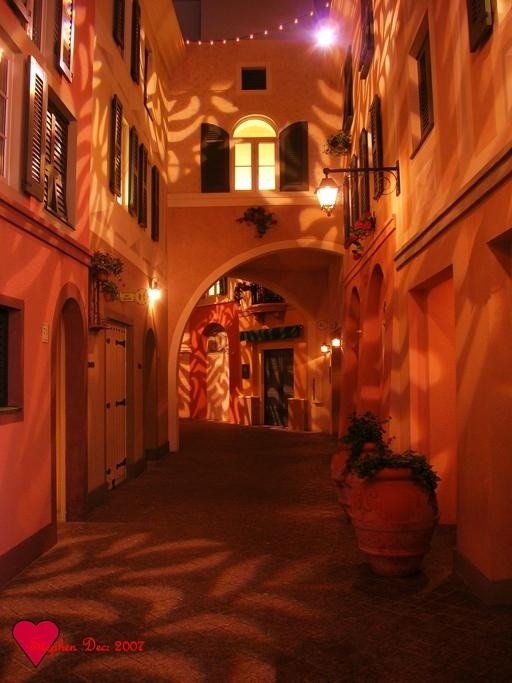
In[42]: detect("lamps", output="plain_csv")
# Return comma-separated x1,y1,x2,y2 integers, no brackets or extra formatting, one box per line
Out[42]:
148,273,162,301
315,159,401,218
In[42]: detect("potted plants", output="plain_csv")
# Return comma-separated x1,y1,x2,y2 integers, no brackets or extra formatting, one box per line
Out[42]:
330,410,393,517
355,449,439,576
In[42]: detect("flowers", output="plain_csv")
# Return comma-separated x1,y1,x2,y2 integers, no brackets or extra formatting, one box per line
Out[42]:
343,208,377,260
